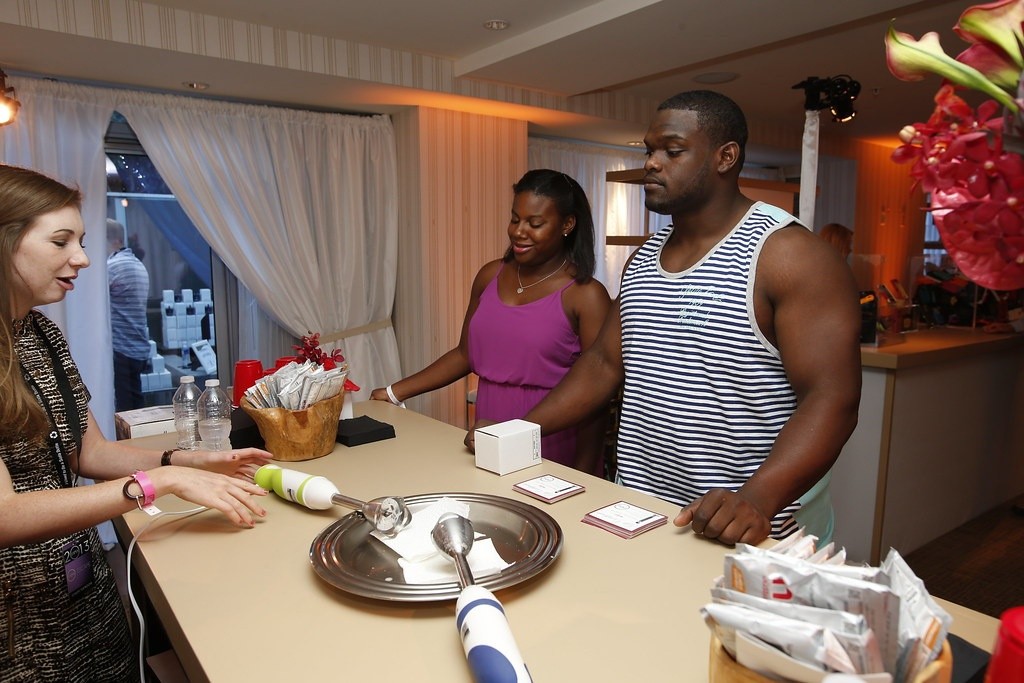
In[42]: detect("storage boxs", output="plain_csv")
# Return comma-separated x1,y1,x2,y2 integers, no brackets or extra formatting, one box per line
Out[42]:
472,416,543,477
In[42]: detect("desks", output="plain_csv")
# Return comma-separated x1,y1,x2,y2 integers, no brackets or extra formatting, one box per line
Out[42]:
101,399,1023,683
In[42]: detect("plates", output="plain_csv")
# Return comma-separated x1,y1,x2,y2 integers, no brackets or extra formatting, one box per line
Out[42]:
308,491,564,603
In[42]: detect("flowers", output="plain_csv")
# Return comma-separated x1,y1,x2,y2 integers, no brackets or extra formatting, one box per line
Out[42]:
882,0,1024,294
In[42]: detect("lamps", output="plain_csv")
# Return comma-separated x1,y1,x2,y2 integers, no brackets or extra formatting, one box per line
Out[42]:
790,74,863,125
0,67,24,128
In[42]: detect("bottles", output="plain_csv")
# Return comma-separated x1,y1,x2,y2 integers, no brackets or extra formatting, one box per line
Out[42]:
197,379,232,453
173,376,203,453
180,339,191,370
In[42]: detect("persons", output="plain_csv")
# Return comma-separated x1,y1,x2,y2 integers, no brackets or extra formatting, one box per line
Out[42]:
0,160,276,683
100,213,154,420
819,222,856,324
461,89,864,550
368,167,615,484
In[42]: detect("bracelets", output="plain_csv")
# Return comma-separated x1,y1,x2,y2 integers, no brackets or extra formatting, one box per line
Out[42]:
160,447,182,466
122,468,160,517
385,383,408,412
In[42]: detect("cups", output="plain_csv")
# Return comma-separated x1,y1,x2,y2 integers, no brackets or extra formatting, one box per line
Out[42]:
232,357,337,407
